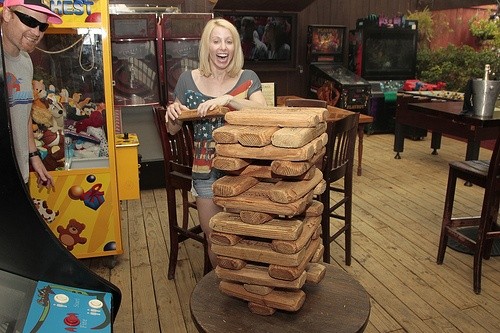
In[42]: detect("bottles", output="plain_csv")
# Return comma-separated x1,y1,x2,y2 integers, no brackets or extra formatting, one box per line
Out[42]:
482,65,497,80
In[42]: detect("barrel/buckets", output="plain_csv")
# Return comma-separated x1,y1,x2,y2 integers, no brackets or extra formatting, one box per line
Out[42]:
471,78,500,118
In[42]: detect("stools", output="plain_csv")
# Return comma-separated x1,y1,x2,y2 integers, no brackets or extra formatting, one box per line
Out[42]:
284,113,361,267
153,106,212,277
437,135,500,295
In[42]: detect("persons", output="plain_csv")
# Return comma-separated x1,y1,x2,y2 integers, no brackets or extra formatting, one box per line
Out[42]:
164,18,267,267
239,17,291,61
0,0,62,194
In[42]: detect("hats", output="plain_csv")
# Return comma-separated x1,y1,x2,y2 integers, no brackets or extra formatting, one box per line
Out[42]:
3,0,63,24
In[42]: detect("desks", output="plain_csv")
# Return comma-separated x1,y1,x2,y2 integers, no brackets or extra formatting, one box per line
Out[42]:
277,95,374,175
189,262,372,333
394,94,500,187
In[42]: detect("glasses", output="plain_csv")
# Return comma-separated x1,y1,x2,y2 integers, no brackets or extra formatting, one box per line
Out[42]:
14,10,49,32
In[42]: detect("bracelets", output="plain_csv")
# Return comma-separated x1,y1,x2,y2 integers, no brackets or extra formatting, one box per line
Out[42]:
30,149,42,159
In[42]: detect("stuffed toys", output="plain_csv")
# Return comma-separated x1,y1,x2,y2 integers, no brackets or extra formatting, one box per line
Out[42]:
30,79,105,171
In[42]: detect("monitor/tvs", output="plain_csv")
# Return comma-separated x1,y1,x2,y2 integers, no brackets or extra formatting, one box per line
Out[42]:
362,28,417,79
209,9,298,71
308,25,346,56
